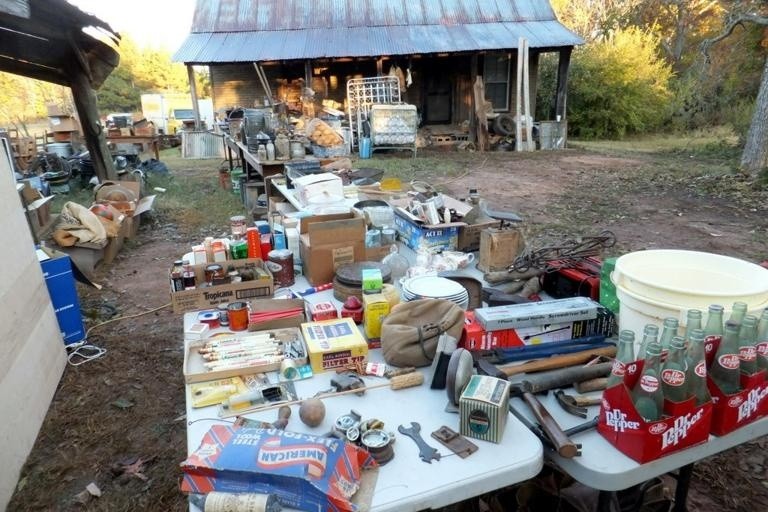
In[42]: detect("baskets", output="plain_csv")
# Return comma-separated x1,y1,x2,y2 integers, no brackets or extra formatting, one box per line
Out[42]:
245,132,276,153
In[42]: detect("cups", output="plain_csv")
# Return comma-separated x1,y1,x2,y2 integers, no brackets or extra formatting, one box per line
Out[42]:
264,95,270,106
556,114,561,122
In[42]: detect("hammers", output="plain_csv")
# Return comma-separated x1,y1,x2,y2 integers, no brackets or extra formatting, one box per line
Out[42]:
480,276,542,308
476,345,617,458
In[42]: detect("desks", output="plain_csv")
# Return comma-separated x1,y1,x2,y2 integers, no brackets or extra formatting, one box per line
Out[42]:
226,136,245,173
181,252,543,512
105,136,160,161
235,140,341,181
271,178,358,214
438,251,768,512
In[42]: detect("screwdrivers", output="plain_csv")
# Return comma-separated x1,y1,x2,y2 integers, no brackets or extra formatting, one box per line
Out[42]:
223,393,281,410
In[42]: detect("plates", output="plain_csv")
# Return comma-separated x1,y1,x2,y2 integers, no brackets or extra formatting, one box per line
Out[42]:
402,275,470,311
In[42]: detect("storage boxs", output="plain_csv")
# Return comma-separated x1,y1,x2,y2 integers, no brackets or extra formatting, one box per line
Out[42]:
293,172,344,207
384,193,474,254
89,205,125,263
96,180,156,240
299,214,365,288
108,116,155,137
169,258,273,315
459,221,501,253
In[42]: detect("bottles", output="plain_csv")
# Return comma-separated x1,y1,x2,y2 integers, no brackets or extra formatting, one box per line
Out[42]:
258,144,266,162
606,301,767,420
220,389,265,414
266,140,276,162
241,268,255,280
229,232,248,260
170,260,197,292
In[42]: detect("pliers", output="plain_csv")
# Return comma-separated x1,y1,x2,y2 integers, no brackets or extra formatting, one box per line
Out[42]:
469,335,617,367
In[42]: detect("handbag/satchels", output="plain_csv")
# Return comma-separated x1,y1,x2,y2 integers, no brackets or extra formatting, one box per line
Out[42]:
381,298,465,366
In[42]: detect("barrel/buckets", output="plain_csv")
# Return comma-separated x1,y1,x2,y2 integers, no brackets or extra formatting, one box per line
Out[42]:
227,108,245,141
231,167,243,194
540,120,568,150
609,248,768,364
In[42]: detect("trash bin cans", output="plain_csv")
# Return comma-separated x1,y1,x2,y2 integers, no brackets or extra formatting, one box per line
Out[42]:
44,141,72,158
113,116,127,129
79,159,96,190
183,120,206,131
